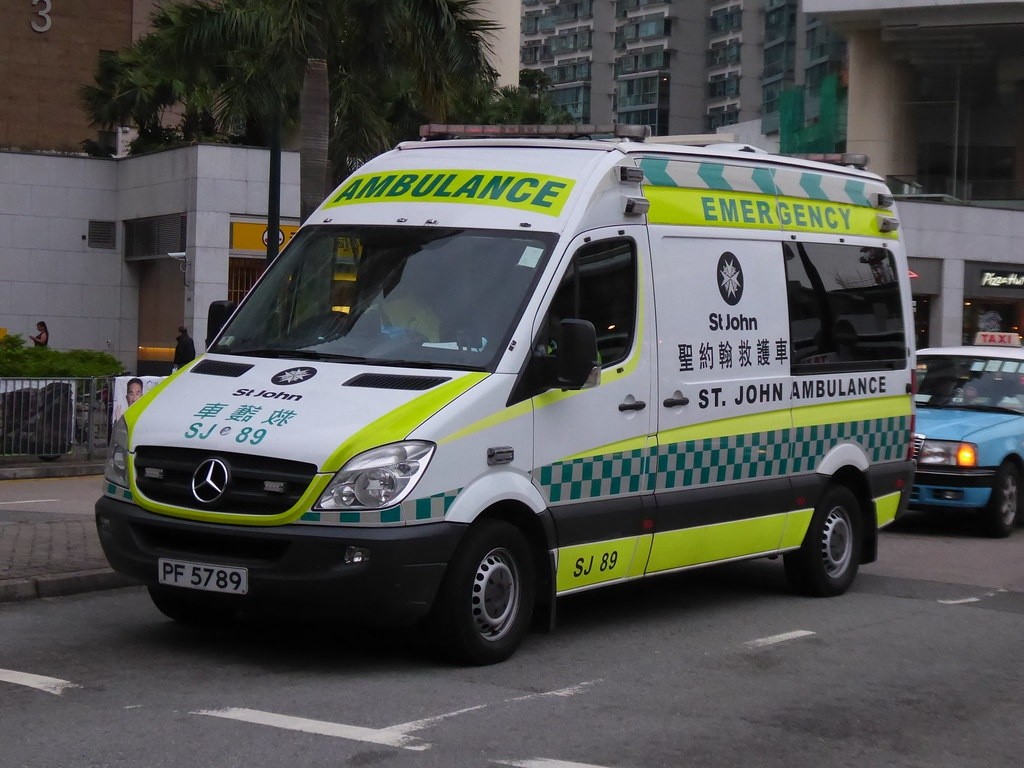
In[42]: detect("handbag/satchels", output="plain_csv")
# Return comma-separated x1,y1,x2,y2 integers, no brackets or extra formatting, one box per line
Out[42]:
172,367,177,373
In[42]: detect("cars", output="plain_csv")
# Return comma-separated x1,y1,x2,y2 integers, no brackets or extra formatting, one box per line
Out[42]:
905,330,1024,539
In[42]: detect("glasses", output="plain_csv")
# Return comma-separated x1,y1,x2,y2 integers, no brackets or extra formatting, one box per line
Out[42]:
127,393,142,398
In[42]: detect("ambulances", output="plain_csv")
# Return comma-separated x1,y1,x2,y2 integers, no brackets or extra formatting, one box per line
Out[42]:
90,123,925,667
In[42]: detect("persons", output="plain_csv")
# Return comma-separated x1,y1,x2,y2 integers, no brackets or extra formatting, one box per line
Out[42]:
536,297,603,365
114,378,143,426
172,326,195,375
29,321,49,347
964,380,981,397
832,320,869,372
326,235,439,342
